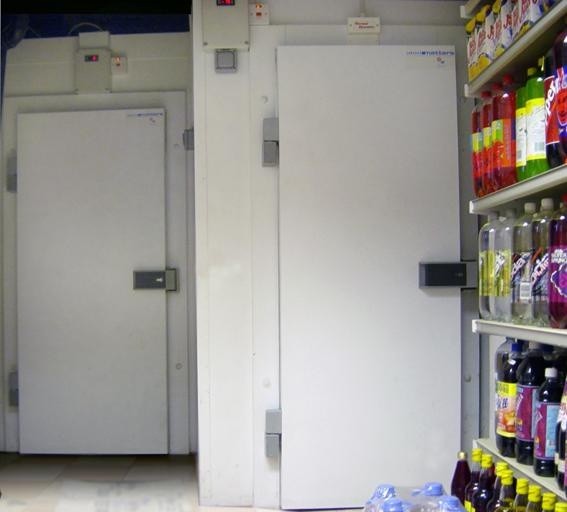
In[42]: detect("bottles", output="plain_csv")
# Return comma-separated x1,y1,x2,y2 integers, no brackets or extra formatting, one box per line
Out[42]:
361,483,465,512
493,338,567,495
452,448,567,511
469,24,567,197
479,197,567,330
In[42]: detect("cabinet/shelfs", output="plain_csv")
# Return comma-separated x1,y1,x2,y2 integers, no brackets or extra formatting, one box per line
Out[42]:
456,1,566,511
